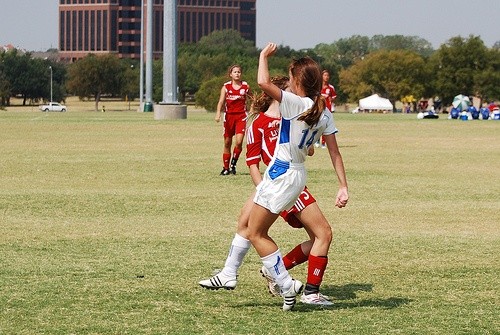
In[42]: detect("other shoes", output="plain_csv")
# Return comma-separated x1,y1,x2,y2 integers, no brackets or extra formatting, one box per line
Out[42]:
315,143,319,147
321,144,325,148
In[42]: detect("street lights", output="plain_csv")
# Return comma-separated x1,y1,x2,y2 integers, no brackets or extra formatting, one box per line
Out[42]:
49,66,53,102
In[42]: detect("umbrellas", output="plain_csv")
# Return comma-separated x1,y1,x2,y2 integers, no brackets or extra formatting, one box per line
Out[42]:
452,94,470,112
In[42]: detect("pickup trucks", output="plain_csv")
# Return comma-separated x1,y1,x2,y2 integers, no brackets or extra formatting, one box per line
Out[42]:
39,102,67,112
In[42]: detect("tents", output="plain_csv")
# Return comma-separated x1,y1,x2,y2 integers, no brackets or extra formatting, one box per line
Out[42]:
359,94,393,114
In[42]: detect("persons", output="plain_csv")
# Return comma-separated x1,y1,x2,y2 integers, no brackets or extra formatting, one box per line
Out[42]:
245,74,335,306
214,64,254,176
450,103,500,120
433,95,442,113
315,69,338,149
197,43,349,311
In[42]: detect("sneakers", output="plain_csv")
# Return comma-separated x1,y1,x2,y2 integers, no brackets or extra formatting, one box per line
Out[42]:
280,278,303,312
230,158,236,175
220,167,230,175
197,272,237,290
260,266,280,296
299,292,334,306
